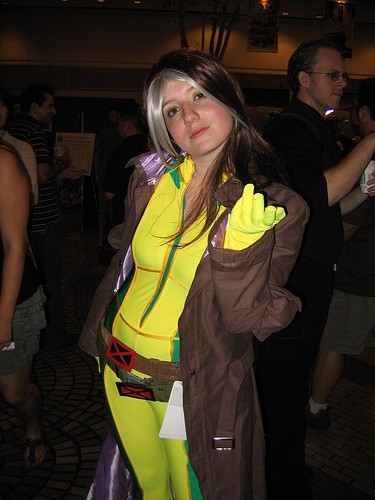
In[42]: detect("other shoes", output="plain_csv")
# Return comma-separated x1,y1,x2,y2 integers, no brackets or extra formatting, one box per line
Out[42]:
306,406,330,432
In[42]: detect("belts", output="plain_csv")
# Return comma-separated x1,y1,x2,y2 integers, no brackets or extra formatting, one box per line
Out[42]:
100,315,185,381
97,325,173,402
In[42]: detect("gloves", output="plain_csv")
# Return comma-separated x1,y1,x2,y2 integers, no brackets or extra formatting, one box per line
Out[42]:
228,183,287,234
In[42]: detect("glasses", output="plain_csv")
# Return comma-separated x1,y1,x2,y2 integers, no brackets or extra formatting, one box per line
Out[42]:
304,71,349,82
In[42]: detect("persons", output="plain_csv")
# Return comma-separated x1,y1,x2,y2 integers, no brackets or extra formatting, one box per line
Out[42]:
0,141,51,472
104,106,151,268
260,37,374,481
14,82,66,321
305,76,374,432
79,48,311,500
0,88,43,268
91,100,133,253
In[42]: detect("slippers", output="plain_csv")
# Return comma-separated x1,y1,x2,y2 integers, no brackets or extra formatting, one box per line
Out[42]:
24,438,47,471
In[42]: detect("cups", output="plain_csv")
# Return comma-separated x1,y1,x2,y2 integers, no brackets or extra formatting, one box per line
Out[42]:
358,152,375,193
53,146,65,158
